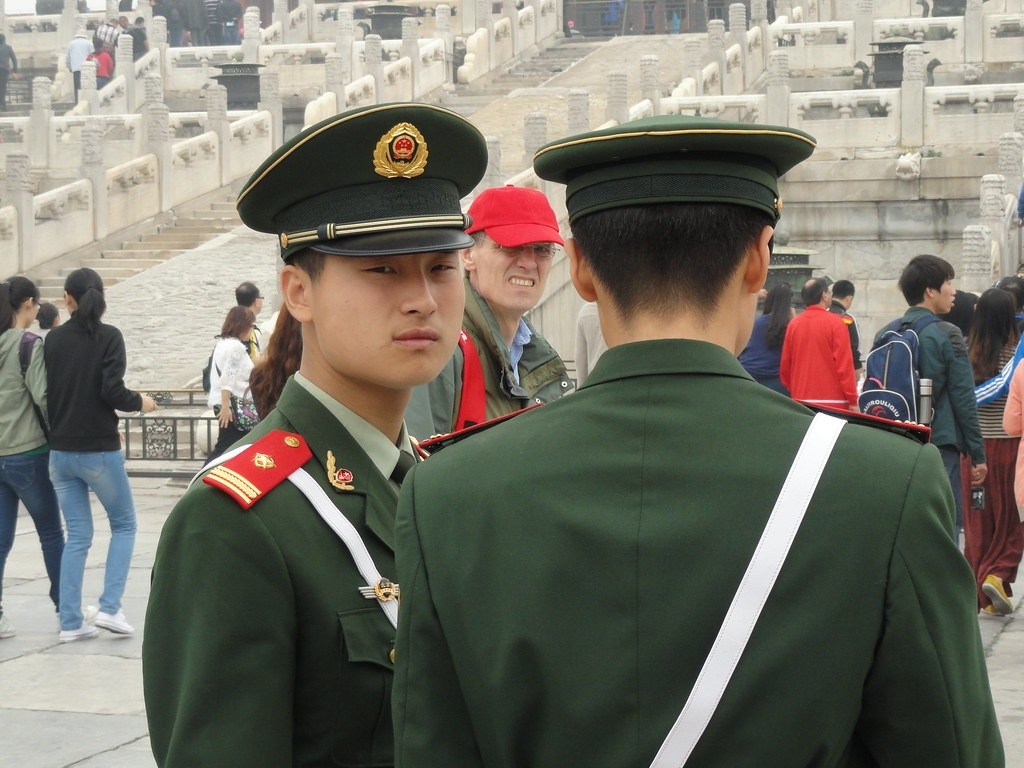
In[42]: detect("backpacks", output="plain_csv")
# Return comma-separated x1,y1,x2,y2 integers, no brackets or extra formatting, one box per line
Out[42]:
858,313,941,431
202,334,222,392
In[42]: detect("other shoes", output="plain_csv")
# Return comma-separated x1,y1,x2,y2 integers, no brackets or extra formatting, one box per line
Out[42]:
979,604,1007,619
981,573,1013,614
0,621,16,638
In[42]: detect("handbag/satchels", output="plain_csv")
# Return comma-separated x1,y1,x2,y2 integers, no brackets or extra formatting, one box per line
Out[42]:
170,8,182,23
228,394,264,431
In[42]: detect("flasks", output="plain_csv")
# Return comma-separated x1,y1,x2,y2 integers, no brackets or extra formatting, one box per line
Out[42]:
919,378,933,424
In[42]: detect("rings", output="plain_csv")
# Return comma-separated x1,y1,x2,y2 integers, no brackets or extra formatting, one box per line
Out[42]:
976,476,980,480
976,472,980,476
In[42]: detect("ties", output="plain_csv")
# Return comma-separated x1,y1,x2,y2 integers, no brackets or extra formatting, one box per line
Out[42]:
389,450,415,483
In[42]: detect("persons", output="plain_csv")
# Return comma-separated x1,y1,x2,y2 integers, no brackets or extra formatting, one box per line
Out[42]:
0,276,99,638
872,254,1024,618
248,300,303,421
738,277,863,412
389,115,1007,768
143,102,485,768
118,0,242,48
44,268,158,643
0,33,17,111
403,185,574,463
37,303,61,346
203,281,265,465
65,15,149,106
574,301,607,389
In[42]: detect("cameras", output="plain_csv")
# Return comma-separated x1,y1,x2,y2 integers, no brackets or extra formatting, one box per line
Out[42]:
970,487,985,511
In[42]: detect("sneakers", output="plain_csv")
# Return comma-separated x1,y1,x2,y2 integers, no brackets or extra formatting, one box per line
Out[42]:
57,621,97,642
93,608,132,634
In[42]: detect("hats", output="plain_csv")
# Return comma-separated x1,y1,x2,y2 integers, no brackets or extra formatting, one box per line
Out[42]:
532,115,818,226
75,28,87,38
235,102,487,256
463,183,566,248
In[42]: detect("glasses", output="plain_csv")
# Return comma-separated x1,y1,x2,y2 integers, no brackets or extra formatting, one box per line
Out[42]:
473,234,561,258
256,295,266,302
22,296,43,311
